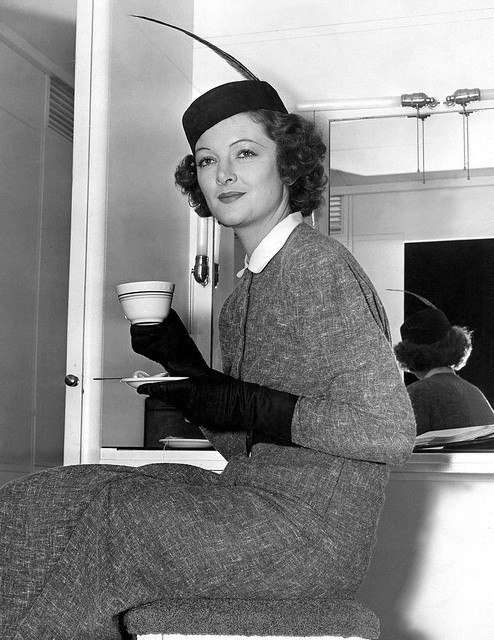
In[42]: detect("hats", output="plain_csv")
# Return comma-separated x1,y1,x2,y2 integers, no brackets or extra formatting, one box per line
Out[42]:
130,12,290,155
385,288,452,344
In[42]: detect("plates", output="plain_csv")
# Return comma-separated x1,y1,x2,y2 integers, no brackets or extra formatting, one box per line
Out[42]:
121,377,189,389
159,439,213,448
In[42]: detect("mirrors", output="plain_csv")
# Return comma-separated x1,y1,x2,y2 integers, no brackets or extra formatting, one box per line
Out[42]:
311,111,494,474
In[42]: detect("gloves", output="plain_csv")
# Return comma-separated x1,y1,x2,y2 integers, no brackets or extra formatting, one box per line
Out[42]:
137,362,299,445
130,309,208,382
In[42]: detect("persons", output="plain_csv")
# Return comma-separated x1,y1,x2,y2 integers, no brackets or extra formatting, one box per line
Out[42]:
1,80,417,640
395,289,493,430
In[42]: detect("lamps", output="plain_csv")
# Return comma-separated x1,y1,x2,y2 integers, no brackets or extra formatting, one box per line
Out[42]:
294,92,435,113
214,215,222,288
446,88,494,106
190,216,209,286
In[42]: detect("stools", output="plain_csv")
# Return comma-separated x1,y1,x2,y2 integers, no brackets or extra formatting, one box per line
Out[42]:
124,599,380,640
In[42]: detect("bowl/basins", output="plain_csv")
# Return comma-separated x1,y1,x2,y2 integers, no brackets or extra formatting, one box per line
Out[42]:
116,281,175,325
